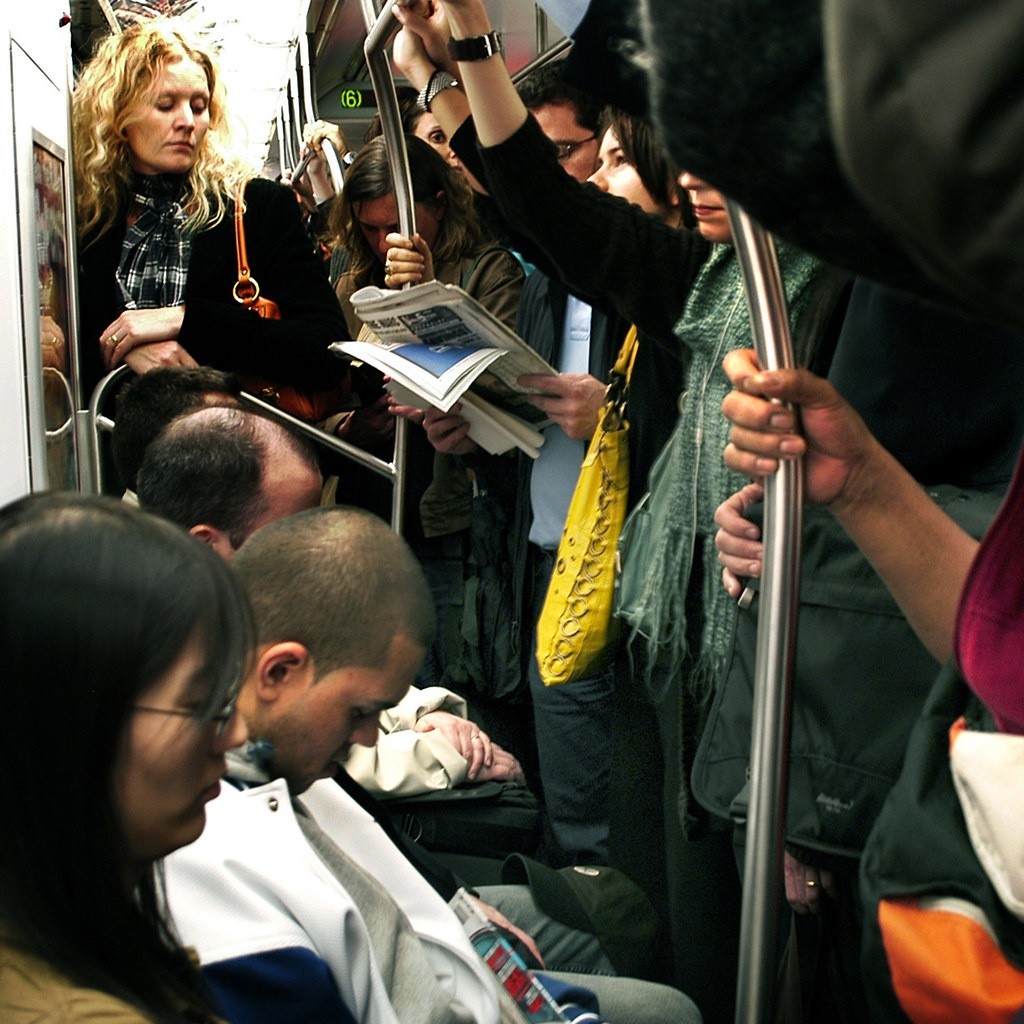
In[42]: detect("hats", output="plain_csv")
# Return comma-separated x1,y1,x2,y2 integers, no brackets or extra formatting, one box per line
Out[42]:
500,852,659,976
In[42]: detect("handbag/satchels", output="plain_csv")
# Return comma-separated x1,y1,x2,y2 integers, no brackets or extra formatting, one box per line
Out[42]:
378,780,539,857
532,325,641,684
696,476,1005,859
232,176,355,422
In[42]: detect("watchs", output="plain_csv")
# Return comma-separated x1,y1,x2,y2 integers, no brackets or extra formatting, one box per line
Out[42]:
416,73,459,111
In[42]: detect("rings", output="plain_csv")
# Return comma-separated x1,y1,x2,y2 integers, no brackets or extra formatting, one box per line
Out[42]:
112,334,119,344
805,880,817,888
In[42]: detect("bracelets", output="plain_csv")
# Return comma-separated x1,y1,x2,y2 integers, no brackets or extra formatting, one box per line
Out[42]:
425,68,444,113
446,30,503,62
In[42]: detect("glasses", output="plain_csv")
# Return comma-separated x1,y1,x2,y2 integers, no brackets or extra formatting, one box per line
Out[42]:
556,136,596,165
133,706,237,740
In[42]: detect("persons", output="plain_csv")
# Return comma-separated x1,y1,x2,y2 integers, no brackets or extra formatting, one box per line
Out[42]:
66,21,351,502
640,0,1024,1024
250,83,611,759
423,0,844,1024
153,509,707,1024
133,396,550,903
0,490,261,1024
39,225,68,378
